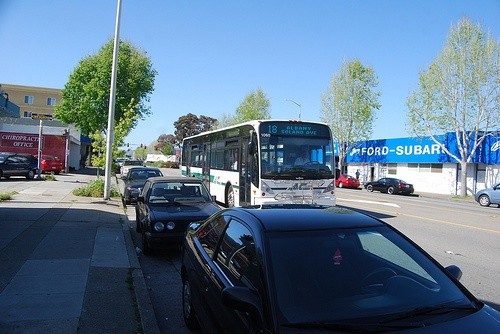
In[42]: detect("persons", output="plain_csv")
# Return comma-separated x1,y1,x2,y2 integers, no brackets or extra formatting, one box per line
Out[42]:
355,170,360,179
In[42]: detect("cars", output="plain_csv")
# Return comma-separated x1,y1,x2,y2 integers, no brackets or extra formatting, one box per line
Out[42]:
130,177,223,255
335,175,361,189
181,207,500,334
364,178,414,196
115,157,164,206
0,152,63,180
475,181,500,207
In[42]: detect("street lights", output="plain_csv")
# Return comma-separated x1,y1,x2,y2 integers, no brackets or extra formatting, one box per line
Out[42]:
283,99,301,120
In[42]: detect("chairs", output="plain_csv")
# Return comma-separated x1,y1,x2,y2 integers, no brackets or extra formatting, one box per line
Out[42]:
153,188,164,197
148,172,155,176
182,186,196,197
132,172,139,177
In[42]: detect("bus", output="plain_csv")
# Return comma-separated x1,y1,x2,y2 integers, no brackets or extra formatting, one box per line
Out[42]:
182,120,339,209
